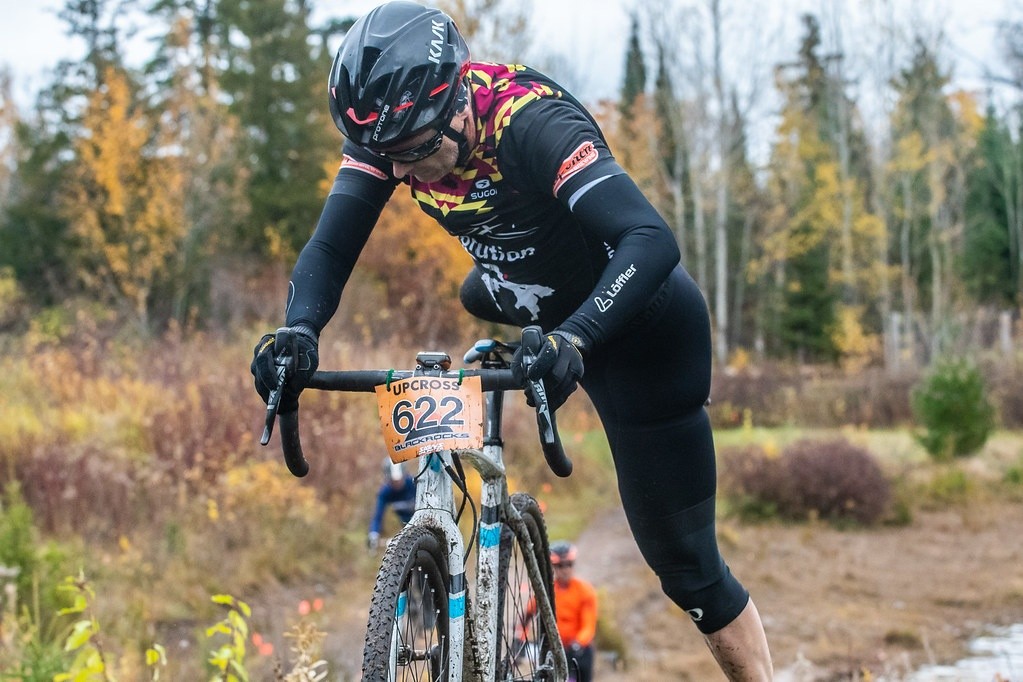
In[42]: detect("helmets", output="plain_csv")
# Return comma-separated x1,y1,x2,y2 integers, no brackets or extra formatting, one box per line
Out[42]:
327,1,472,149
547,540,577,564
383,455,407,481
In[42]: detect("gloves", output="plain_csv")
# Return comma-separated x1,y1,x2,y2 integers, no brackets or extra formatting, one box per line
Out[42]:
250,331,318,418
511,639,527,658
510,333,585,416
568,642,585,660
368,530,380,549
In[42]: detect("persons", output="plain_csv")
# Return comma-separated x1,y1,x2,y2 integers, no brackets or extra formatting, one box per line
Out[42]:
250,2,775,682
511,539,599,682
365,455,436,636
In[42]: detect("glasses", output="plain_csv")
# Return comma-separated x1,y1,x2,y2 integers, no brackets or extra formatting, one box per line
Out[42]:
363,80,462,164
553,562,574,568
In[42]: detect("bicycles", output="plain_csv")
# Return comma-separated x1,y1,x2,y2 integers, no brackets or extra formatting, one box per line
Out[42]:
260,325,586,682
